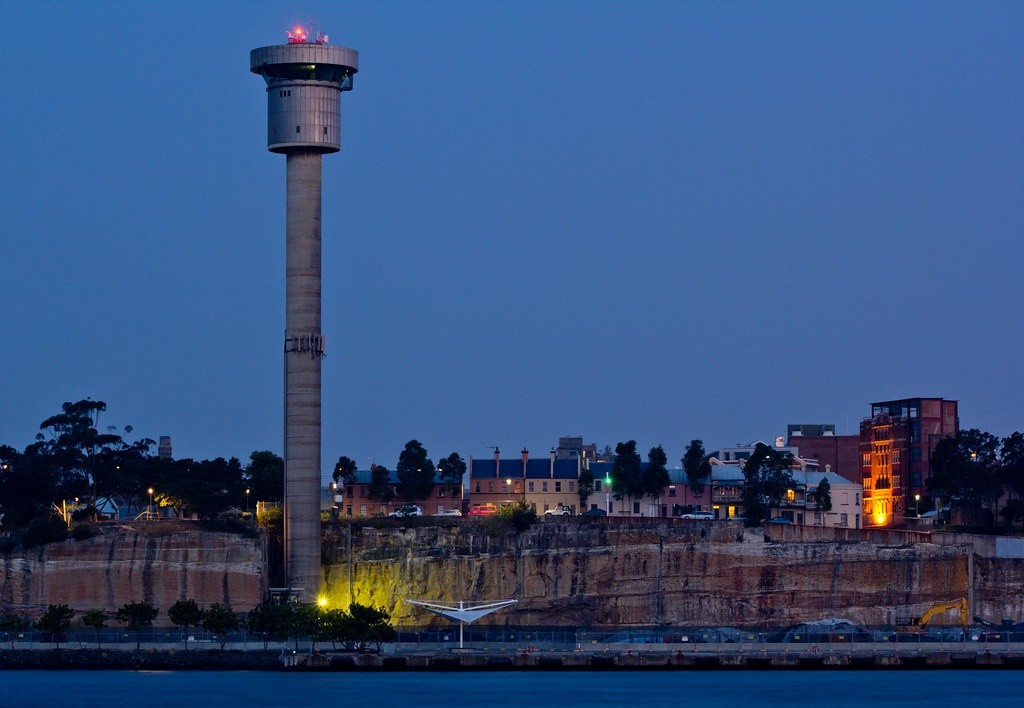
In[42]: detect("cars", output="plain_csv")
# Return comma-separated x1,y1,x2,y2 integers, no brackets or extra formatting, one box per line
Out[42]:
432,509,462,516
681,511,715,521
764,517,790,524
578,508,607,516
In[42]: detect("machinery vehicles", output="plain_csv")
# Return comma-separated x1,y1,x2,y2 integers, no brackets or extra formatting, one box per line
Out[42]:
889,597,971,643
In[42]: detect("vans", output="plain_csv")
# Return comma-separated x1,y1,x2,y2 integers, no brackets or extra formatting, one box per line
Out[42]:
468,505,496,516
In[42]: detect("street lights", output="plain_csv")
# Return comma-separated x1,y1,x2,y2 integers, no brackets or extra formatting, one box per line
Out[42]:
246,489,250,512
75,497,79,510
506,478,512,507
148,488,153,520
915,494,920,518
605,472,611,516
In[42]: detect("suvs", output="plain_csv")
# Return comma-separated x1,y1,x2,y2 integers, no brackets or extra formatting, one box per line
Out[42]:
544,505,572,517
388,505,422,518
